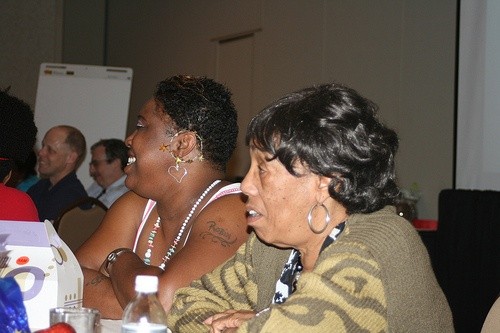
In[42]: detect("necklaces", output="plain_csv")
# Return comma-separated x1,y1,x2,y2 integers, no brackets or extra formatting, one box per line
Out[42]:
144,179,221,271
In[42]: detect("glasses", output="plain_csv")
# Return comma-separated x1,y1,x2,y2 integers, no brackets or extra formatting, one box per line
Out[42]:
92,159,107,166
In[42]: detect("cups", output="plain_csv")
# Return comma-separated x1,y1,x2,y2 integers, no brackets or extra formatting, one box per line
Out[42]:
48,307,98,333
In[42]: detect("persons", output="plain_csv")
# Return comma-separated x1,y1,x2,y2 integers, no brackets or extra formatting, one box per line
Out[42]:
164,82,455,333
85,138,131,209
74,74,253,321
0,96,40,222
25,125,89,222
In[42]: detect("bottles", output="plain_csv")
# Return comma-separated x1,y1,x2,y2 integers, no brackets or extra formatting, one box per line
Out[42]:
120,274,168,333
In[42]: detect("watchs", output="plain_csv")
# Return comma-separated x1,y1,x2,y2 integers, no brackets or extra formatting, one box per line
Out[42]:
105,248,131,275
